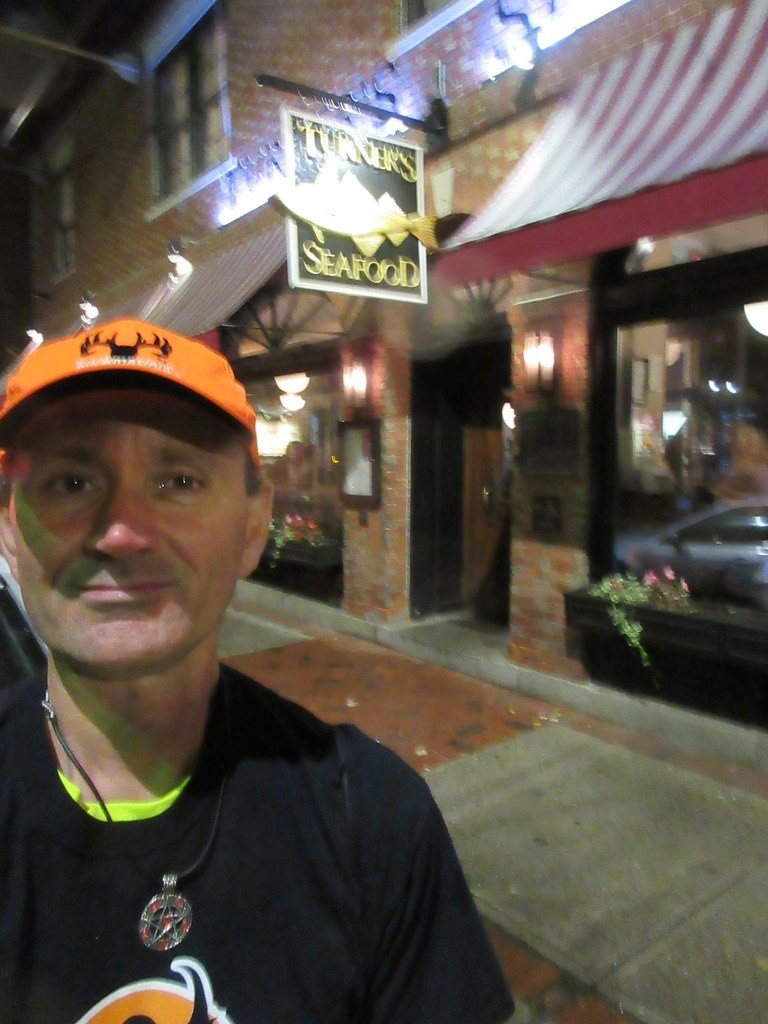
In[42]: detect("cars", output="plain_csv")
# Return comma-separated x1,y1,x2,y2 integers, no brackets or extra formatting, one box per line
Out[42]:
612,494,768,613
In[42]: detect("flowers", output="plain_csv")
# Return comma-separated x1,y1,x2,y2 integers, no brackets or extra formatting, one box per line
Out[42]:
587,563,694,689
268,513,324,569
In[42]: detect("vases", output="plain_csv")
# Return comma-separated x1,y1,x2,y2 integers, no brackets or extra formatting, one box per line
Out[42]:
562,580,767,734
249,533,343,601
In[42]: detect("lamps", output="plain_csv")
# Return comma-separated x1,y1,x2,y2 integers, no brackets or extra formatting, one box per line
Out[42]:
523,322,557,392
337,333,370,407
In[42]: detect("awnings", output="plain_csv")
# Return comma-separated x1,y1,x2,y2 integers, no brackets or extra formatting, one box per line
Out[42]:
430,2,768,293
0,221,290,397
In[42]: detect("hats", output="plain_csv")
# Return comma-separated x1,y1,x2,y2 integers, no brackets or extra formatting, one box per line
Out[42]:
0,318,264,490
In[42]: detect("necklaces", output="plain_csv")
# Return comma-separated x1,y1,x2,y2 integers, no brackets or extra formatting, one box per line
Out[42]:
37,683,238,952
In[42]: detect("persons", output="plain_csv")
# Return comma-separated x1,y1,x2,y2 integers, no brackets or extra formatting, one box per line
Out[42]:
0,320,516,1024
